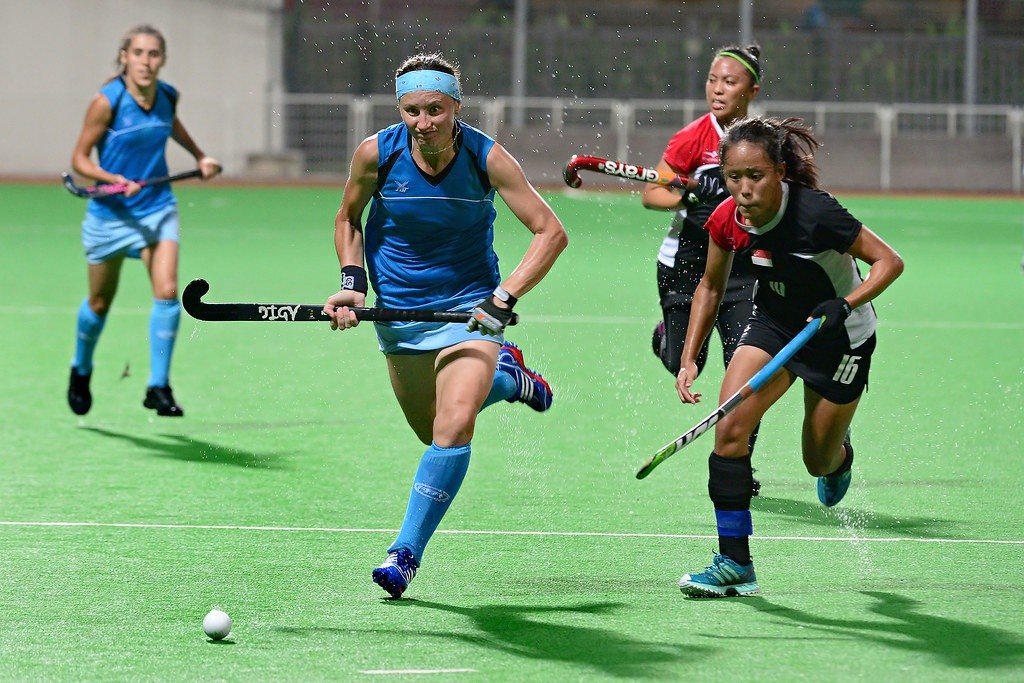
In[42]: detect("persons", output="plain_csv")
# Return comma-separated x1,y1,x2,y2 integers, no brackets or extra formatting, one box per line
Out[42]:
671,115,905,596
639,43,761,498
67,23,223,418
318,55,569,601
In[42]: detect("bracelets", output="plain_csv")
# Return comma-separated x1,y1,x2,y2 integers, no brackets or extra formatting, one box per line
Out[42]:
339,264,370,297
492,284,519,308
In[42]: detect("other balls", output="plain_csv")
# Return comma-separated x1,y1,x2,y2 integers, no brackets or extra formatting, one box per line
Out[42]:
202,608,233,640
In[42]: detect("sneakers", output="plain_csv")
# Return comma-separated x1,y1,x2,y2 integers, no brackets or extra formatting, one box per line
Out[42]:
68,368,92,415
373,548,417,599
497,340,553,412
818,427,851,507
679,549,760,598
144,385,184,417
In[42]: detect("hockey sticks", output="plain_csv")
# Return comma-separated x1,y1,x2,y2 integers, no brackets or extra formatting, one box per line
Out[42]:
181,277,520,329
61,163,222,199
634,314,823,480
563,154,701,194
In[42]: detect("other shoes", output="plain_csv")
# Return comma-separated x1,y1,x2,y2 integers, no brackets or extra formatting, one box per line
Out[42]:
653,322,666,357
